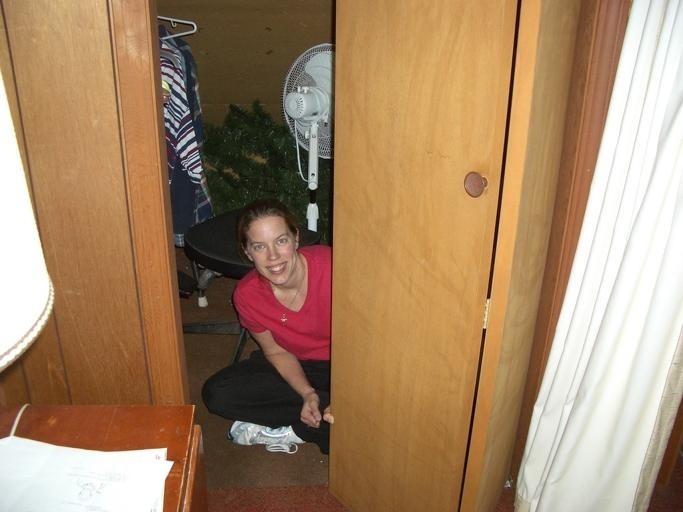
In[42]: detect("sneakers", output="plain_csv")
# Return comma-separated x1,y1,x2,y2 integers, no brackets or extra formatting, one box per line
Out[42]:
226,419,306,454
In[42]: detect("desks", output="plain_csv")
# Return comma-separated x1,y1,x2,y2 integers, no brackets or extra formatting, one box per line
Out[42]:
1,402,202,511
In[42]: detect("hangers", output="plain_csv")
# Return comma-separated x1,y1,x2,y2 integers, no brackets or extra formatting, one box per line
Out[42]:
158,13,198,42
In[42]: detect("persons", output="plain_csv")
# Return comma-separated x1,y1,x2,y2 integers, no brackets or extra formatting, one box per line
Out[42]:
200,198,334,456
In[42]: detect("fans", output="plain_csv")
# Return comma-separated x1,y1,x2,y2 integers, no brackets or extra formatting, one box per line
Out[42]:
278,41,332,234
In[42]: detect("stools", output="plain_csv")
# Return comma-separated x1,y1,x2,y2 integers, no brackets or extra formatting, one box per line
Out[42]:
182,194,321,375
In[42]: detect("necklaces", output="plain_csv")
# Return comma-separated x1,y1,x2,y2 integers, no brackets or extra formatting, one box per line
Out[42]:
260,255,305,327
